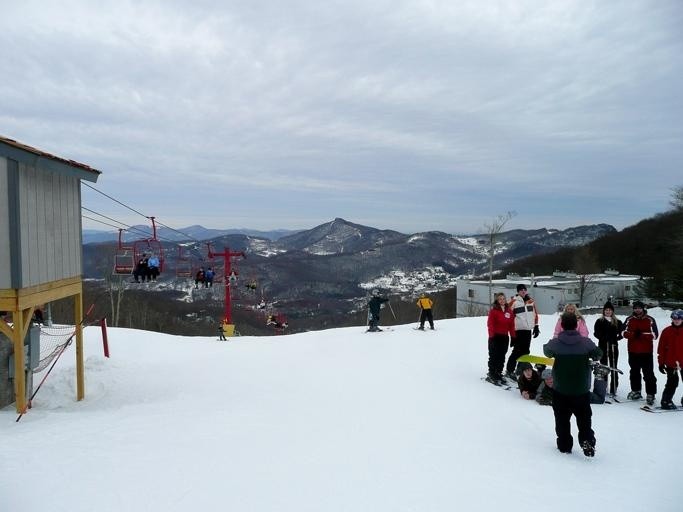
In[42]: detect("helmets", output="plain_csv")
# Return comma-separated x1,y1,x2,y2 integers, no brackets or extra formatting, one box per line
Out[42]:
541,369,552,379
671,309,682,319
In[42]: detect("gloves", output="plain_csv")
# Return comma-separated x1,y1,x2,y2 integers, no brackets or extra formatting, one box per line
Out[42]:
532,325,540,338
510,337,516,346
659,365,667,374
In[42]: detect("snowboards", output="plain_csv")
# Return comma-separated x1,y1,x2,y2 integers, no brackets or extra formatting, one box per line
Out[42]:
589,359,625,374
516,355,554,365
642,405,683,413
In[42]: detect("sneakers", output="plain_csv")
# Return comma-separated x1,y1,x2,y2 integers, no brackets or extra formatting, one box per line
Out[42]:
660,400,677,410
130,280,158,283
641,399,655,410
485,361,532,385
627,390,643,400
582,440,595,457
366,327,435,332
593,367,610,376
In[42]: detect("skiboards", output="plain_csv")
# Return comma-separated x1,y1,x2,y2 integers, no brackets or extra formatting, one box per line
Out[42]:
480,371,519,390
614,398,654,409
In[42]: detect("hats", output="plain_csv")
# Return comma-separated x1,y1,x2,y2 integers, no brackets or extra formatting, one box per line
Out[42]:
560,311,578,330
632,302,646,308
604,300,614,310
517,284,527,292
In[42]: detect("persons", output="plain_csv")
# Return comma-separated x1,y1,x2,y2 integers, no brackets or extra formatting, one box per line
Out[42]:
133,253,148,283
487,284,683,457
205,268,216,288
229,268,238,276
365,289,389,331
195,267,205,289
218,324,226,340
417,293,434,330
147,254,160,282
